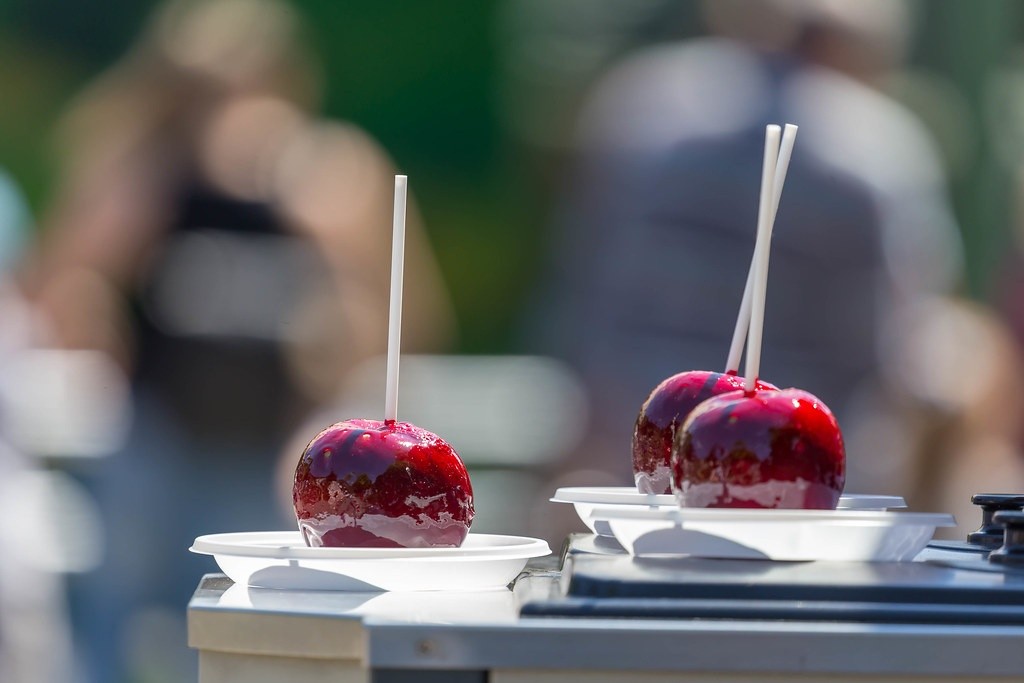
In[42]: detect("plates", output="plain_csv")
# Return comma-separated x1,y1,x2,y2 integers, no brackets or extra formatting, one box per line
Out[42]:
592,508,958,559
551,487,905,538
189,531,550,591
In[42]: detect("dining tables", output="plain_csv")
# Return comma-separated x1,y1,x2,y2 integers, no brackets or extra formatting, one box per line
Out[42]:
186,540,1024,682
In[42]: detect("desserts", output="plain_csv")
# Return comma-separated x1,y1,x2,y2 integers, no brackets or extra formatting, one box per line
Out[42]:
629,122,845,512
291,174,474,549
